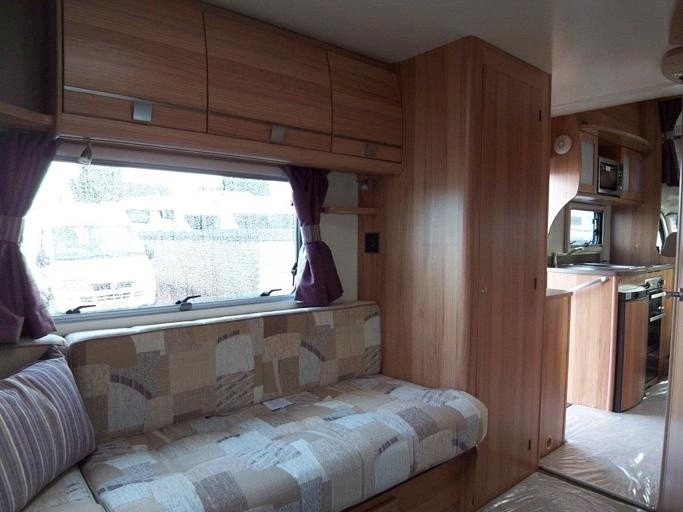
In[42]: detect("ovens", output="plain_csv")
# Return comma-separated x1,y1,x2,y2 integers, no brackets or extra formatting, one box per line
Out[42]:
644,277,666,392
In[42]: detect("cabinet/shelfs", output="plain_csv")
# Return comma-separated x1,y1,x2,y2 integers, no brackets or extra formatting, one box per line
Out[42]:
659,269,675,381
539,288,573,459
613,286,649,412
360,35,552,512
30,1,405,177
571,125,653,207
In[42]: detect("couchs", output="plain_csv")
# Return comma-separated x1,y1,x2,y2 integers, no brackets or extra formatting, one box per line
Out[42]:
0,300,488,511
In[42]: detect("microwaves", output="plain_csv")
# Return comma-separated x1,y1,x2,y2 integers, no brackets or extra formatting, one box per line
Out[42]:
598,157,624,197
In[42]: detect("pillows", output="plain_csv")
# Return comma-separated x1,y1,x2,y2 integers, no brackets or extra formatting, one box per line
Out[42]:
0,345,96,511
